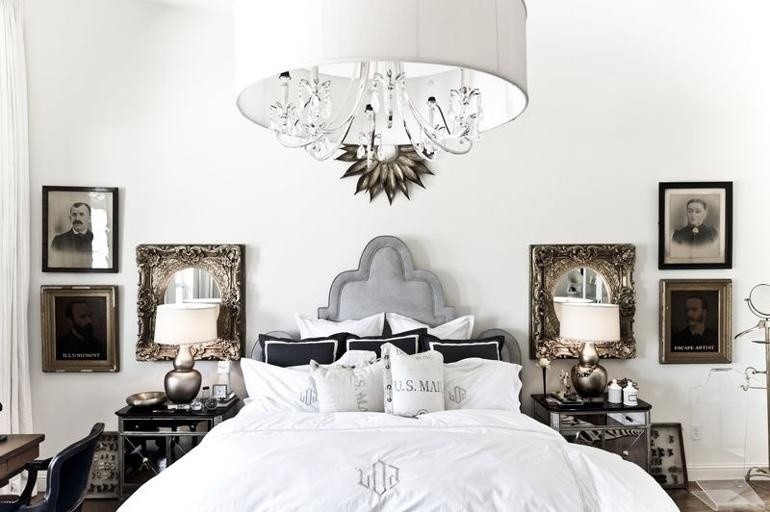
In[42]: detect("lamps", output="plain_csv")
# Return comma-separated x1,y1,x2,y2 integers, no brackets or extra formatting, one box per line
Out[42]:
551,295,590,317
185,298,222,307
559,302,622,399
152,303,219,405
233,0,529,169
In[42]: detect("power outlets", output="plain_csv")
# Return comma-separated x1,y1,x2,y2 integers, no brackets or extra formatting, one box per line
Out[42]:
217,361,231,374
689,424,702,440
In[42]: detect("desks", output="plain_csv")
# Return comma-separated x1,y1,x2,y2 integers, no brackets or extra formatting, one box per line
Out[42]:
0,434,45,482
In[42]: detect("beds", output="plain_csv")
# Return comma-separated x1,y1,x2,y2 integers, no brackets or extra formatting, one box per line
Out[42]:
115,235,682,512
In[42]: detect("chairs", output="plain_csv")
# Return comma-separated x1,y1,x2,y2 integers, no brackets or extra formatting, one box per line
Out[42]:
0,422,105,512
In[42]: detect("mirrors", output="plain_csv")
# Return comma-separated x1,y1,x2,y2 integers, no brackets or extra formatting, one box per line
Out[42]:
135,243,247,363
527,244,636,361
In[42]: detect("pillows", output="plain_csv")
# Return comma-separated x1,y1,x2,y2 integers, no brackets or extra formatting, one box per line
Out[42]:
240,314,523,421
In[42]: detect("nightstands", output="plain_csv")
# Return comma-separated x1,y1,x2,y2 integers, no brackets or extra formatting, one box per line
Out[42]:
115,399,240,502
530,391,653,475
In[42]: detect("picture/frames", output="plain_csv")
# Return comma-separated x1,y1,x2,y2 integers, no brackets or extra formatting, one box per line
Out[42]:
41,185,118,273
86,431,119,500
658,280,734,365
650,422,689,490
40,284,120,375
656,180,733,271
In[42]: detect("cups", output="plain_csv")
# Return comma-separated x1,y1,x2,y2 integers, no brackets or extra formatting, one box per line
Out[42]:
623,382,638,406
202,386,210,402
608,379,623,403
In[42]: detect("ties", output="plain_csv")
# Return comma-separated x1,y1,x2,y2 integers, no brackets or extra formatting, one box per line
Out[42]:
694,334,701,341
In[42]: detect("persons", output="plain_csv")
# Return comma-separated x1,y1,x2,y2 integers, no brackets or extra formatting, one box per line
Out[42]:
671,199,718,247
671,297,718,352
50,202,94,253
56,301,107,360
